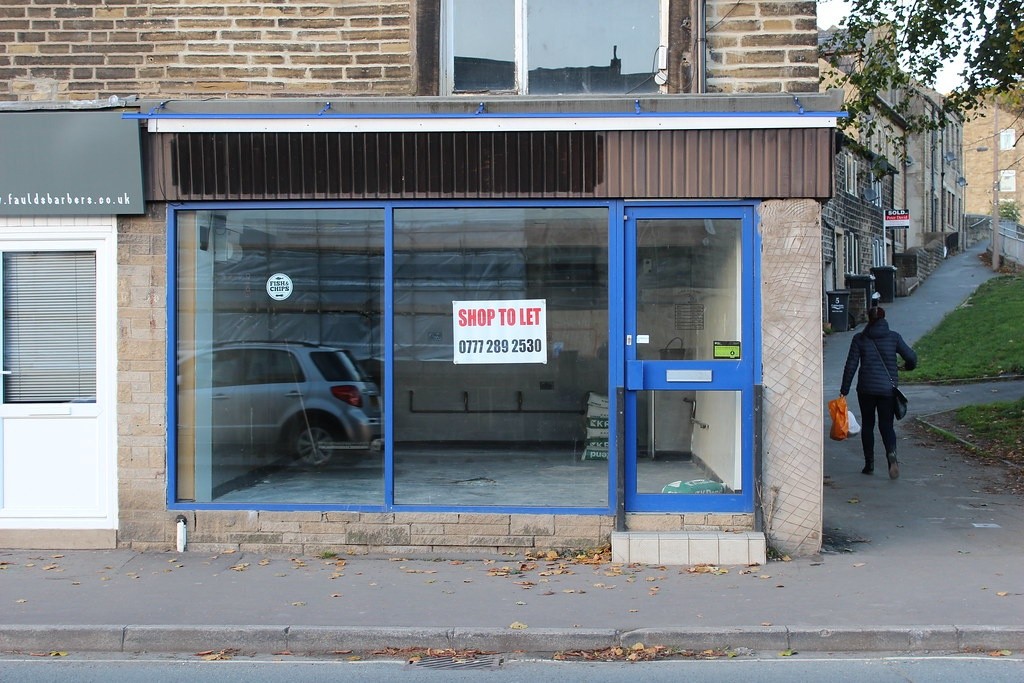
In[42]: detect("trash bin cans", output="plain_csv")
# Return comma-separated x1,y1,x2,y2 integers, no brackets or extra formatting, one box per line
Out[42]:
844,274,876,312
872,297,880,307
870,265,898,303
826,290,851,332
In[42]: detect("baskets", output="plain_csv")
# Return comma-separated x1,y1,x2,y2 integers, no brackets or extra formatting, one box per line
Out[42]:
658,337,686,360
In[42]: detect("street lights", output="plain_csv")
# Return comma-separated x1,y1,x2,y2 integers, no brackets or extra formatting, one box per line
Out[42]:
961,145,991,251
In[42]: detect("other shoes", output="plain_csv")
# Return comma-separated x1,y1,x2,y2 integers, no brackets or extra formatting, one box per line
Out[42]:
862,463,874,473
887,449,899,479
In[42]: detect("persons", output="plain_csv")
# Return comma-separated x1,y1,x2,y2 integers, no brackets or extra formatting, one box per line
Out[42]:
840,307,918,478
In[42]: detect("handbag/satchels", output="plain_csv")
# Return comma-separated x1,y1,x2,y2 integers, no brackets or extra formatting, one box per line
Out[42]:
847,411,862,438
828,396,848,441
893,387,908,420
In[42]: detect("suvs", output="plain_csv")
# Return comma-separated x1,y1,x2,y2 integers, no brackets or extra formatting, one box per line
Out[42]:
176,338,384,472
